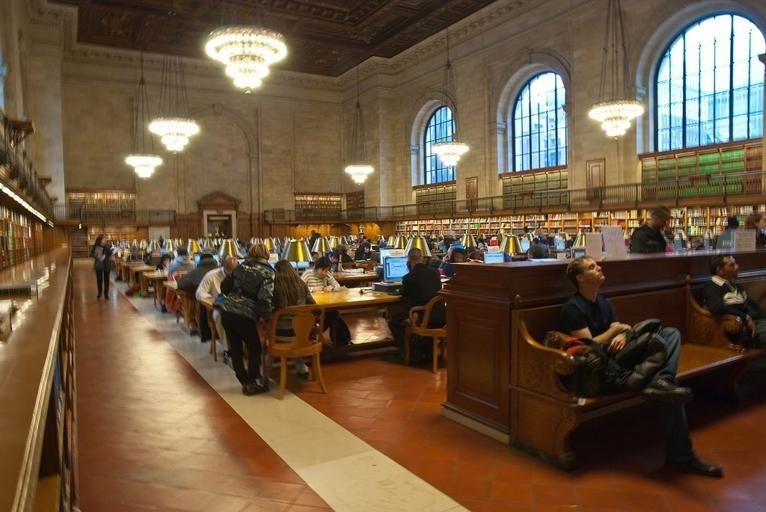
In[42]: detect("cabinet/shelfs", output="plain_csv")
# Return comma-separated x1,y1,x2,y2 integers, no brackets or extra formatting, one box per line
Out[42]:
393,196,765,258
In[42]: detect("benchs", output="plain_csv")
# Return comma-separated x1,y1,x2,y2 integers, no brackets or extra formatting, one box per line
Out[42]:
509,284,709,436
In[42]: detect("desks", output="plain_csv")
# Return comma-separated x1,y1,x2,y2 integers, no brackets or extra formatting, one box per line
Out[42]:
113,257,404,372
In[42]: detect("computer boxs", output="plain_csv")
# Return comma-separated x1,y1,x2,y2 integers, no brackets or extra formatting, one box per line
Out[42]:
372,283,401,295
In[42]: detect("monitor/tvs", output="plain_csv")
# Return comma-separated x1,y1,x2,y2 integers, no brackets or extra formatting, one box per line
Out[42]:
384,257,409,280
288,260,311,269
378,248,405,265
194,251,207,263
483,252,505,266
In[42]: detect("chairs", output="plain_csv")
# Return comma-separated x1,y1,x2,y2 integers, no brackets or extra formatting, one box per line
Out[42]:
405,296,448,373
261,303,328,400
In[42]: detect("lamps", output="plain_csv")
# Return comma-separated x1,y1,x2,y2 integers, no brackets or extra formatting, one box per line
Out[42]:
204,0,289,94
123,9,200,181
431,2,469,168
587,0,646,141
343,50,374,186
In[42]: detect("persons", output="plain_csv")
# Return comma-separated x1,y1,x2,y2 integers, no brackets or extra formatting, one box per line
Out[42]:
703,254,766,351
271,260,321,376
126,231,386,365
429,231,570,277
629,206,671,252
389,245,443,364
215,243,270,397
90,234,114,299
557,255,723,479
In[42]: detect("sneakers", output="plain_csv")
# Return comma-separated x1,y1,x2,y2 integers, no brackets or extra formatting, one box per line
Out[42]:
243,384,268,394
296,364,309,376
665,456,722,476
644,380,690,401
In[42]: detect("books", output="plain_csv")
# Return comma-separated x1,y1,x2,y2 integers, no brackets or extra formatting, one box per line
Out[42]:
417,185,455,211
637,145,762,202
0,204,80,512
669,205,765,250
399,209,652,238
499,172,569,210
292,195,365,217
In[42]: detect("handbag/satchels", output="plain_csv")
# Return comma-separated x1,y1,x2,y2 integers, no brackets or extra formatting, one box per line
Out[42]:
544,331,604,396
